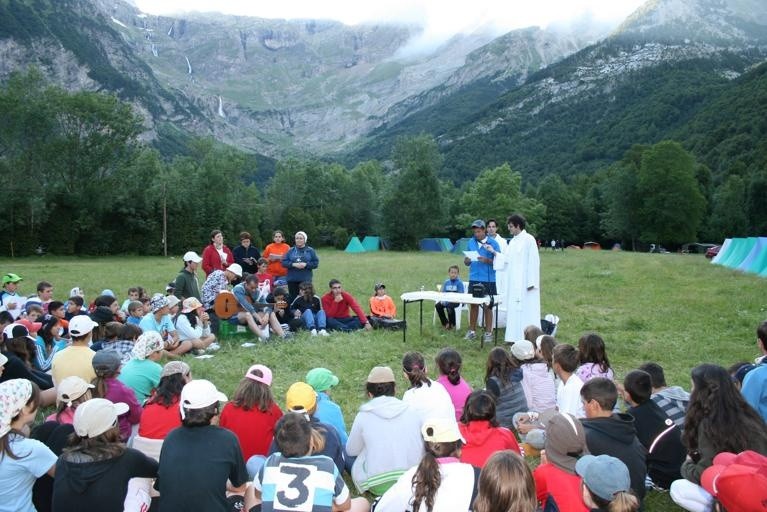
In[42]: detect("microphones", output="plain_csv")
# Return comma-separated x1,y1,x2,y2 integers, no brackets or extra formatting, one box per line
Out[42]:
474,238,485,245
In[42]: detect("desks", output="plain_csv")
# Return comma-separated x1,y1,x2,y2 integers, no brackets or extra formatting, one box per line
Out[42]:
401,290,501,347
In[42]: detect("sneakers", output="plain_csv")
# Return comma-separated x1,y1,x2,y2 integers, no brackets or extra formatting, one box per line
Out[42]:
484,332,494,342
318,329,328,336
310,329,317,336
464,330,477,339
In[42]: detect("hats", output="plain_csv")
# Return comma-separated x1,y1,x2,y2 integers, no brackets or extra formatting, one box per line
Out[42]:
701,465,767,512
376,282,386,290
511,340,535,360
575,454,631,500
181,297,203,313
472,220,486,229
367,366,395,383
2,273,24,288
245,364,272,387
227,262,242,277
167,295,180,309
68,314,98,337
14,319,42,332
305,368,339,391
57,376,96,402
539,408,587,458
183,251,203,263
421,417,467,445
713,451,767,475
0,353,8,367
3,323,37,341
286,381,318,413
73,398,129,438
150,293,172,314
179,379,228,420
296,231,308,243
92,349,120,373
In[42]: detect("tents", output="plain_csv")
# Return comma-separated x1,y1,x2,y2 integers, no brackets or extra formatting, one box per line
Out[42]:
345,236,365,252
362,235,388,251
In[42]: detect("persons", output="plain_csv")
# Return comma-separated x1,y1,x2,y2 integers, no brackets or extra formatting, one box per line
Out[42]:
576,333,614,384
174,251,204,311
201,230,234,281
729,361,754,395
575,454,640,512
638,362,691,432
321,278,372,332
478,218,507,329
0,378,74,512
0,352,8,378
463,219,501,343
752,322,767,364
133,360,192,463
510,339,556,414
435,265,464,330
14,319,66,372
232,232,261,286
370,417,481,512
401,351,455,437
532,413,591,512
219,365,284,465
262,230,290,281
50,315,99,397
0,273,216,360
200,263,242,314
118,331,165,408
473,450,536,512
485,214,541,345
244,413,351,512
434,348,473,420
290,281,329,337
158,379,249,512
266,287,293,324
305,367,349,448
270,382,346,479
512,344,585,451
615,370,687,494
254,258,273,291
578,377,648,502
229,274,289,344
456,390,521,469
369,282,406,329
88,349,143,442
0,323,53,390
536,334,556,372
345,366,423,497
51,398,160,512
523,324,542,347
484,346,528,430
668,364,767,511
281,231,319,302
